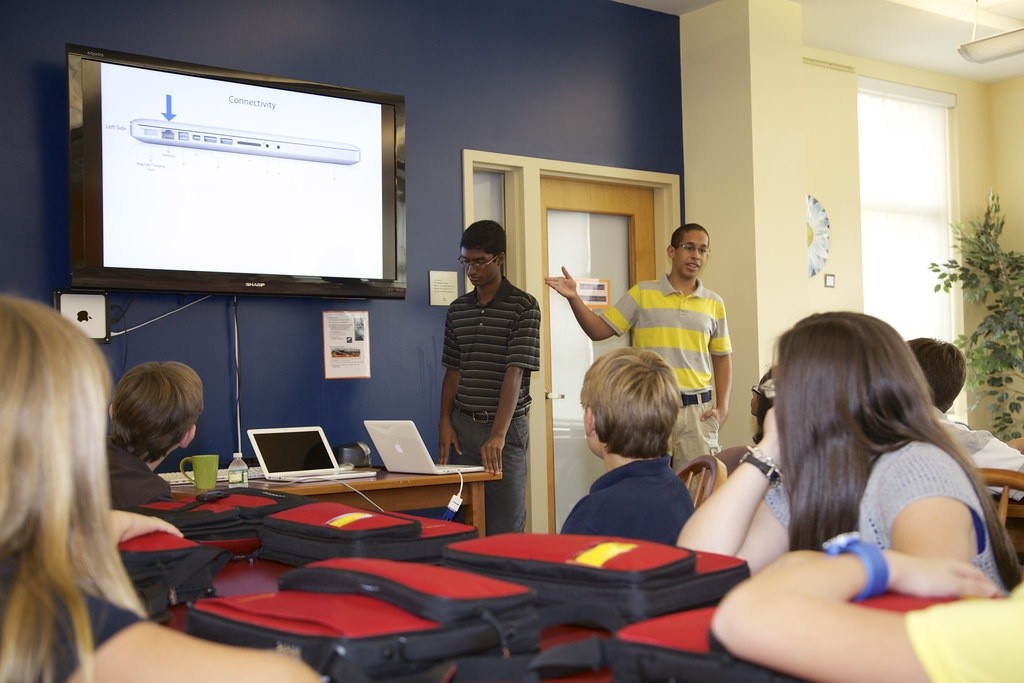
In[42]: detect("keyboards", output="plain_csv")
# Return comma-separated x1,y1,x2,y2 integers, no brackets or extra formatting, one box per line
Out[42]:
159,467,266,484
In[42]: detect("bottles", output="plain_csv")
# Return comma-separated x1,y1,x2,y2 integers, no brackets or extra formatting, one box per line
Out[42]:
228,452,249,488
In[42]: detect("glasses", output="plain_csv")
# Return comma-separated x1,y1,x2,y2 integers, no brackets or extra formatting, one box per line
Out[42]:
677,244,711,255
751,385,763,396
457,251,501,270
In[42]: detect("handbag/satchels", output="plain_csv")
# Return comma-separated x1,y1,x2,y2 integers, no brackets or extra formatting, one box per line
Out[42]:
184,558,542,683
260,499,480,567
115,530,232,616
442,533,751,633
598,605,797,683
129,486,318,570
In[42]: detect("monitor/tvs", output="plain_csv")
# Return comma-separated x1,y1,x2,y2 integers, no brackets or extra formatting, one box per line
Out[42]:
65,44,406,301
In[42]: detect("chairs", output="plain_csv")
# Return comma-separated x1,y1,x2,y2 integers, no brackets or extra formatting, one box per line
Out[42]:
673,453,726,507
973,463,1024,586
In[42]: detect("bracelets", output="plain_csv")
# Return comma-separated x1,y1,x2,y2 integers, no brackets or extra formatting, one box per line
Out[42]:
738,445,782,482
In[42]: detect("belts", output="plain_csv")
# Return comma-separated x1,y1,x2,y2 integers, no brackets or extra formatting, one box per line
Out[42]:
455,405,531,424
681,390,711,405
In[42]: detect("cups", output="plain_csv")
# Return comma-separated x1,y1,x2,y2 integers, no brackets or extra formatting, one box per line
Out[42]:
180,454,219,490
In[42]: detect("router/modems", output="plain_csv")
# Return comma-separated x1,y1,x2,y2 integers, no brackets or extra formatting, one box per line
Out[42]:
53,289,111,344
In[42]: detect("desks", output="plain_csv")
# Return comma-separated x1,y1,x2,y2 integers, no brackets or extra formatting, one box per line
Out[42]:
165,468,499,538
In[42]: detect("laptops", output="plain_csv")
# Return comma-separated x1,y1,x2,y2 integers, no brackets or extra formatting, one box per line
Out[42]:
129,119,362,165
364,419,485,474
247,426,376,483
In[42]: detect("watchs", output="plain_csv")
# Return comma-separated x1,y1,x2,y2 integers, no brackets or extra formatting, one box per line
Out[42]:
822,531,888,600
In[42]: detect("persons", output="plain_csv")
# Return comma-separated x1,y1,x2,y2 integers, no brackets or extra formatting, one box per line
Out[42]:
751,365,775,445
437,220,541,536
710,528,1024,683
558,346,696,546
544,223,733,473
0,294,323,683
106,361,204,511
675,311,1022,594
906,338,1024,501
